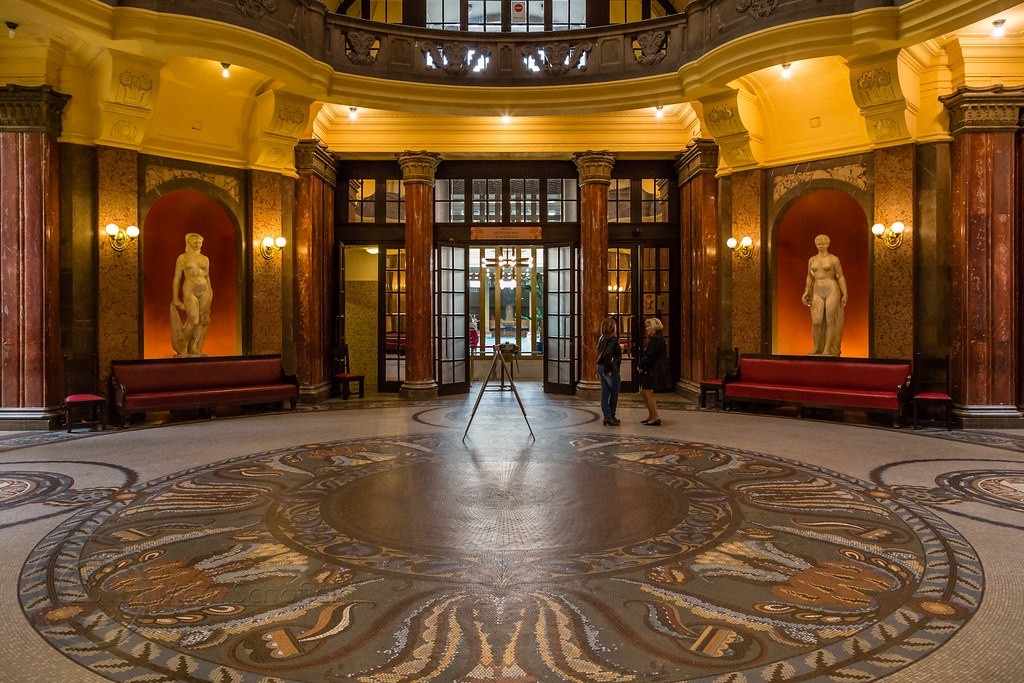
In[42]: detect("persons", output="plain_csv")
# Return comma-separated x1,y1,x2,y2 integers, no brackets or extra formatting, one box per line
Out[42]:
169,233,213,357
637,318,668,426
596,318,622,426
802,234,848,357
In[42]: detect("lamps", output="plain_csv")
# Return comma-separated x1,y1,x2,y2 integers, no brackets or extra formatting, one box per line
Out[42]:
347,108,358,121
221,62,231,77
993,19,1006,37
781,60,790,76
105,223,139,252
481,246,534,289
657,104,663,118
260,236,286,260
6,22,22,38
872,223,905,249
726,236,755,259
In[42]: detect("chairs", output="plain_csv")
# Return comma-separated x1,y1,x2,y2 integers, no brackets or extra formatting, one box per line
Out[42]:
700,347,740,409
912,352,954,430
63,354,107,433
333,344,366,400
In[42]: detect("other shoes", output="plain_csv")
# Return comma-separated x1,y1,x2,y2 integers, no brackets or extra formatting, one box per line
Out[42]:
640,420,650,423
643,419,662,426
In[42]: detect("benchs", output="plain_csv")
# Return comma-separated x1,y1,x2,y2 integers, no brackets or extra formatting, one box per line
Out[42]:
108,353,300,427
724,354,915,426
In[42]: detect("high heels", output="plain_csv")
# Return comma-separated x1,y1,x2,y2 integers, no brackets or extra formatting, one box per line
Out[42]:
603,419,619,426
612,416,621,423
